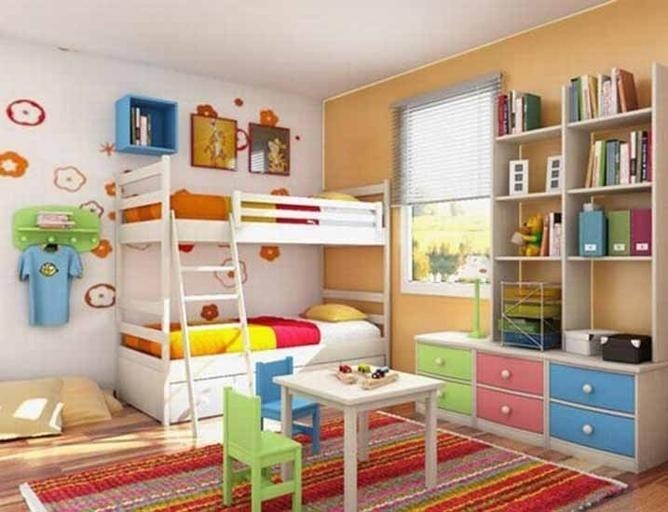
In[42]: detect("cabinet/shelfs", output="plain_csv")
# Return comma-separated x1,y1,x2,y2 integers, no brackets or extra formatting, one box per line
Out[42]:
491,64,668,364
414,330,477,429
114,95,178,156
547,348,668,475
476,328,549,449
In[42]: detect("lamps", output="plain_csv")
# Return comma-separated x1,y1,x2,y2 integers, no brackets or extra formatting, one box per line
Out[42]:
455,255,492,338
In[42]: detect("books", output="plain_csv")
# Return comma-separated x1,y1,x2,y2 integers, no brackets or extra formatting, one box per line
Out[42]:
584,129,653,187
131,106,153,146
36,210,76,229
539,212,562,258
568,67,640,123
497,89,540,137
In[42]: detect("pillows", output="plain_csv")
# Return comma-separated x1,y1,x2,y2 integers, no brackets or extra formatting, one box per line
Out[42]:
310,188,360,201
2,379,63,442
299,304,365,322
62,377,111,426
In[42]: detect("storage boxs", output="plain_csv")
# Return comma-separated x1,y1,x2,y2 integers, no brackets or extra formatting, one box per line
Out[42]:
631,211,653,256
610,211,631,251
579,210,603,257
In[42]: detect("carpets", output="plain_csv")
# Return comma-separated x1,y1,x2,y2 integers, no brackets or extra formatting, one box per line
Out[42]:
20,412,627,512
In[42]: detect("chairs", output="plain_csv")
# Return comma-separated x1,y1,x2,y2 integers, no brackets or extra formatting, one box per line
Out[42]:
224,381,303,512
254,357,322,455
272,363,445,512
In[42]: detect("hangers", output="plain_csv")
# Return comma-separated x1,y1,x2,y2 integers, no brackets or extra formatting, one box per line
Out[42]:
42,242,60,254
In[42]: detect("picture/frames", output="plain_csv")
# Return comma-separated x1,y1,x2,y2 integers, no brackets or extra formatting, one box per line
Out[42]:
190,113,238,171
546,155,561,190
508,158,529,196
248,125,290,175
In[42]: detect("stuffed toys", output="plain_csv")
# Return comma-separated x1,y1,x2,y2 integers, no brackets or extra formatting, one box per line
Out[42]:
517,215,542,258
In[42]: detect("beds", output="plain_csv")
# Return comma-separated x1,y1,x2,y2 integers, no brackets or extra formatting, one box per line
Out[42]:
113,155,394,426
